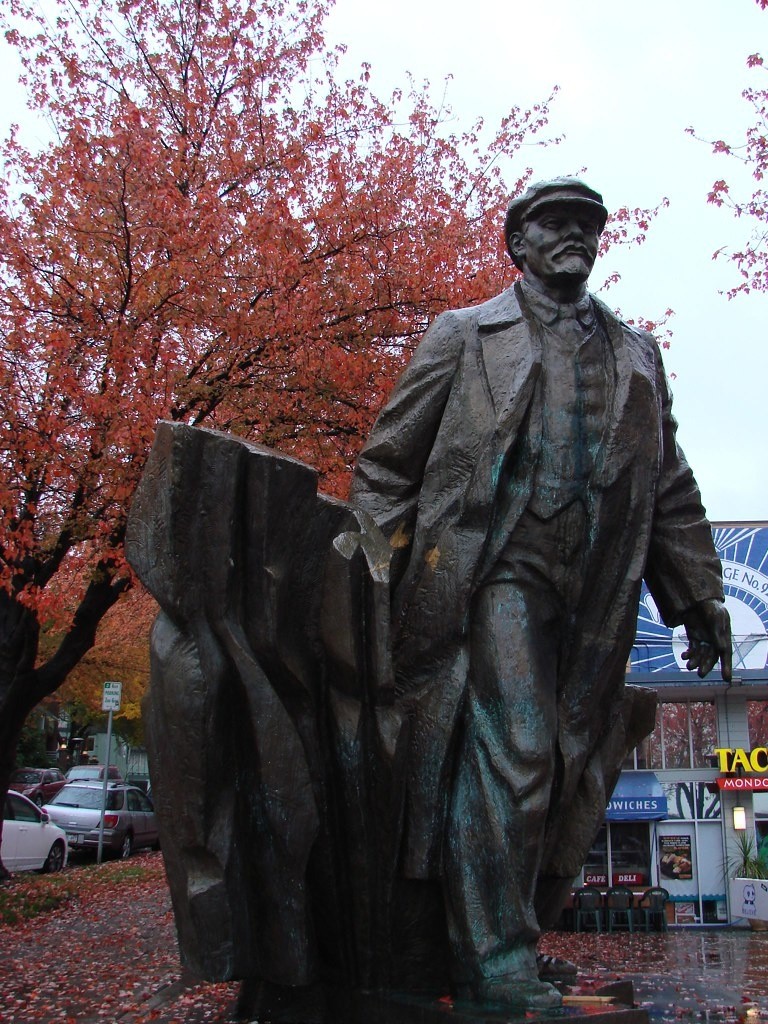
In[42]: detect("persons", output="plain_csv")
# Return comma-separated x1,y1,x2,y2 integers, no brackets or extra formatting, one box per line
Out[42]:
352,178,734,1010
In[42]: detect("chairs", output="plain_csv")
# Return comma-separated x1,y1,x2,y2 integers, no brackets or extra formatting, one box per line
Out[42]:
572,887,603,934
636,887,669,933
604,886,635,932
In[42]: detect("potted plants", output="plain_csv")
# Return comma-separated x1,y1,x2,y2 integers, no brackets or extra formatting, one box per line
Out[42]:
719,830,768,930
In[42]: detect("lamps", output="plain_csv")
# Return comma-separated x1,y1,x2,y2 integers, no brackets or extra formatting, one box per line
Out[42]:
732,806,747,830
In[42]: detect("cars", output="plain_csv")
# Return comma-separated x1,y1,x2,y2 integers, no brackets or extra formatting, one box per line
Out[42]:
64,764,123,783
1,789,69,874
7,766,66,809
41,779,162,862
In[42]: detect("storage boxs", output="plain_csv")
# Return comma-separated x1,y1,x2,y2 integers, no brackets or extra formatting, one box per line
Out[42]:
674,902,697,924
